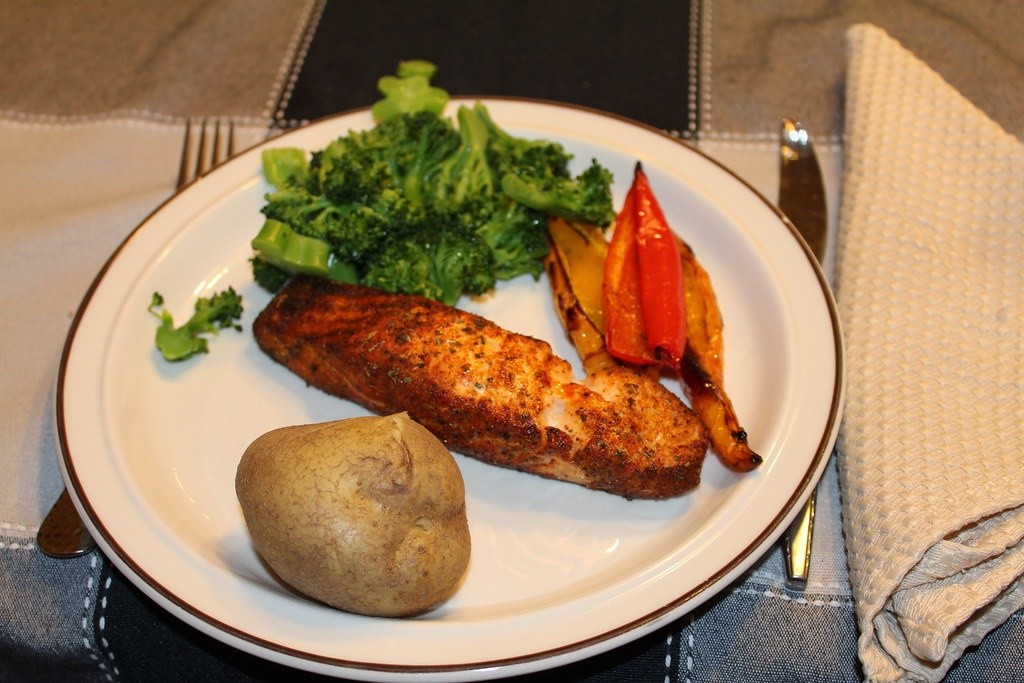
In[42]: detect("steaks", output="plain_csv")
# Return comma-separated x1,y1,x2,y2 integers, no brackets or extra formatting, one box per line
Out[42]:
254,275,711,500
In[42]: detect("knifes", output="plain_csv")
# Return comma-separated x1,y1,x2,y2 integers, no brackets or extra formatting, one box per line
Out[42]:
778,116,828,584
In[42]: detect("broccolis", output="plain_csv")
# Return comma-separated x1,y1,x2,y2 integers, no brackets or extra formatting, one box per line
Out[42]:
143,62,614,362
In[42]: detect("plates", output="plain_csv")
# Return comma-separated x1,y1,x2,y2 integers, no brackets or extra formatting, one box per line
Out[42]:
52,96,847,683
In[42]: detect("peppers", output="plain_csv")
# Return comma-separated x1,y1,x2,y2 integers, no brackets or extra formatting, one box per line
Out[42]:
596,157,689,369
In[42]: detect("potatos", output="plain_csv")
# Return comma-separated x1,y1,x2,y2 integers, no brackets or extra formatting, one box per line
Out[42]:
234,411,471,618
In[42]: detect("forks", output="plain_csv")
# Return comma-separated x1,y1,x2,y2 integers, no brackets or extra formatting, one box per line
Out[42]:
36,117,235,559
